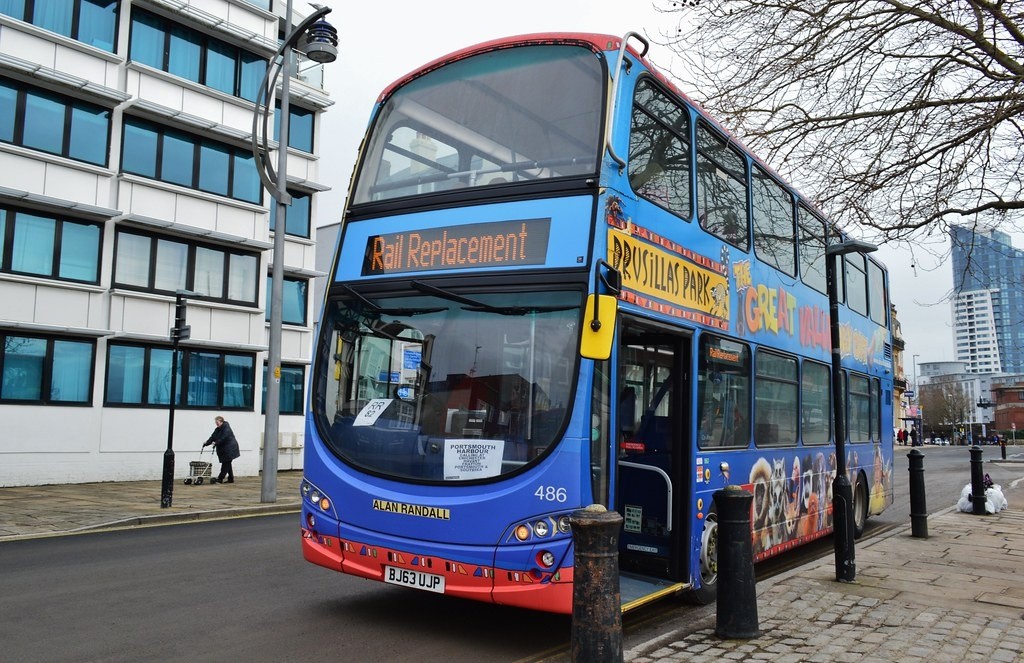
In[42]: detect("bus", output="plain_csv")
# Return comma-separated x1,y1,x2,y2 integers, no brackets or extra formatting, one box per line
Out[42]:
303,30,894,619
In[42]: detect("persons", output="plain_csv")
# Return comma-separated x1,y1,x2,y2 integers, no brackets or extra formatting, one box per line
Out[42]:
902,428,908,446
930,431,936,444
897,428,904,446
940,431,946,446
910,426,916,447
203,416,240,484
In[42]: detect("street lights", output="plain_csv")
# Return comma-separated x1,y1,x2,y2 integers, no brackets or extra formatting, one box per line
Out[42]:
252,1,338,505
913,355,920,392
826,241,878,581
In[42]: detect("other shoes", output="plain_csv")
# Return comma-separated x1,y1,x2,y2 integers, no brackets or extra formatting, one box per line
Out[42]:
216,478,223,484
224,476,233,483
